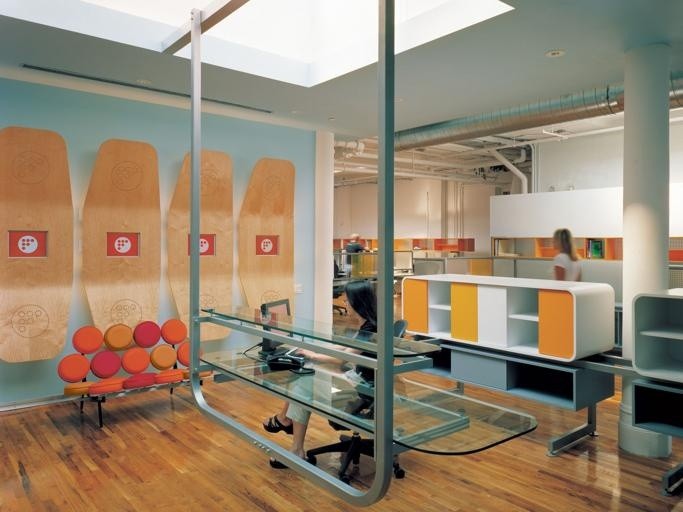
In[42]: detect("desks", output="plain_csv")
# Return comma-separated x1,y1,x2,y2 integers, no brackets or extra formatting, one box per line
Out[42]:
190,304,539,506
399,271,682,498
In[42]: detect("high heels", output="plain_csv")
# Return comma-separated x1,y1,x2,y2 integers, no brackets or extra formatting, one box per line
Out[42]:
263,415,293,434
270,454,285,469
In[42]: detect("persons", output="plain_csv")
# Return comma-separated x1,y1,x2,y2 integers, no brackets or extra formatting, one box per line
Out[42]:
260,277,378,468
334,257,345,275
345,234,372,276
550,229,581,282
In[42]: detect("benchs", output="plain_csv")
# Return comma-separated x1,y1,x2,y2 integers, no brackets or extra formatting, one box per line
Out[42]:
51,320,209,430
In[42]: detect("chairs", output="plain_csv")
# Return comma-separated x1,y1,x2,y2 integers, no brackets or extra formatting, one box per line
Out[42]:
326,318,414,483
